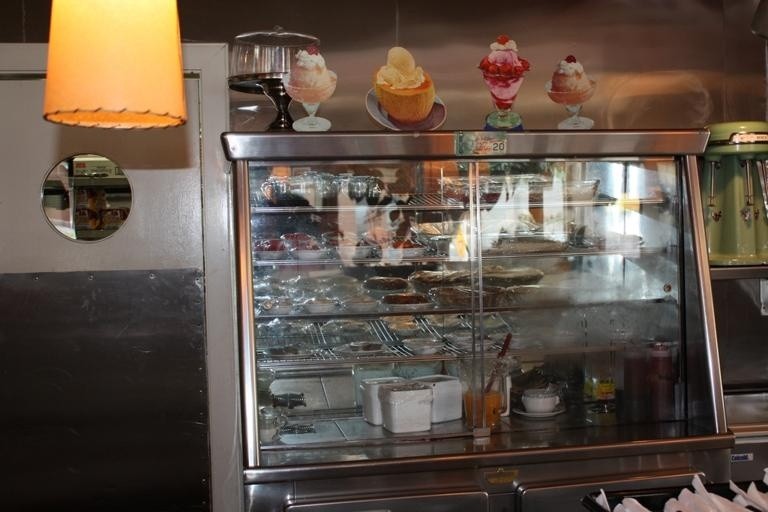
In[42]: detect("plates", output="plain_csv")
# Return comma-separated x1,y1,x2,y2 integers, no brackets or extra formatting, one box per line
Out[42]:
512,408,567,419
363,83,449,132
246,161,495,370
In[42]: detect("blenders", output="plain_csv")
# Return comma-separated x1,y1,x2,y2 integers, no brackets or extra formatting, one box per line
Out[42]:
698,120,768,267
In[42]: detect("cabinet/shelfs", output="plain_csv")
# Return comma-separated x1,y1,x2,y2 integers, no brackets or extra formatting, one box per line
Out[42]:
220,130,768,511
44,155,128,240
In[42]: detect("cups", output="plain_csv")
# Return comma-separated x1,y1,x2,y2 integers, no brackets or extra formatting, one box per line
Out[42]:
461,357,509,433
521,389,560,413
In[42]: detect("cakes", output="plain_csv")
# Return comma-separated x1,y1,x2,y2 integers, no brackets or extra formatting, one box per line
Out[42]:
374,46,435,122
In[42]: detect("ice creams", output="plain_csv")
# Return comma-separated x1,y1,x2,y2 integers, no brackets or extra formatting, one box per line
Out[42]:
548,55,595,106
282,44,337,103
479,35,529,111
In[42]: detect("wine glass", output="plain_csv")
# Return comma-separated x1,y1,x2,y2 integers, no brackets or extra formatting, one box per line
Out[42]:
544,75,596,131
477,54,530,130
282,68,338,133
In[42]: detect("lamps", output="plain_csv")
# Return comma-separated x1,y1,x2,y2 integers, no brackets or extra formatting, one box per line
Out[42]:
43,0,187,131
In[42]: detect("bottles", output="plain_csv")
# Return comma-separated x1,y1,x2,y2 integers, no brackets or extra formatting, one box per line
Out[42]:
622,347,677,424
